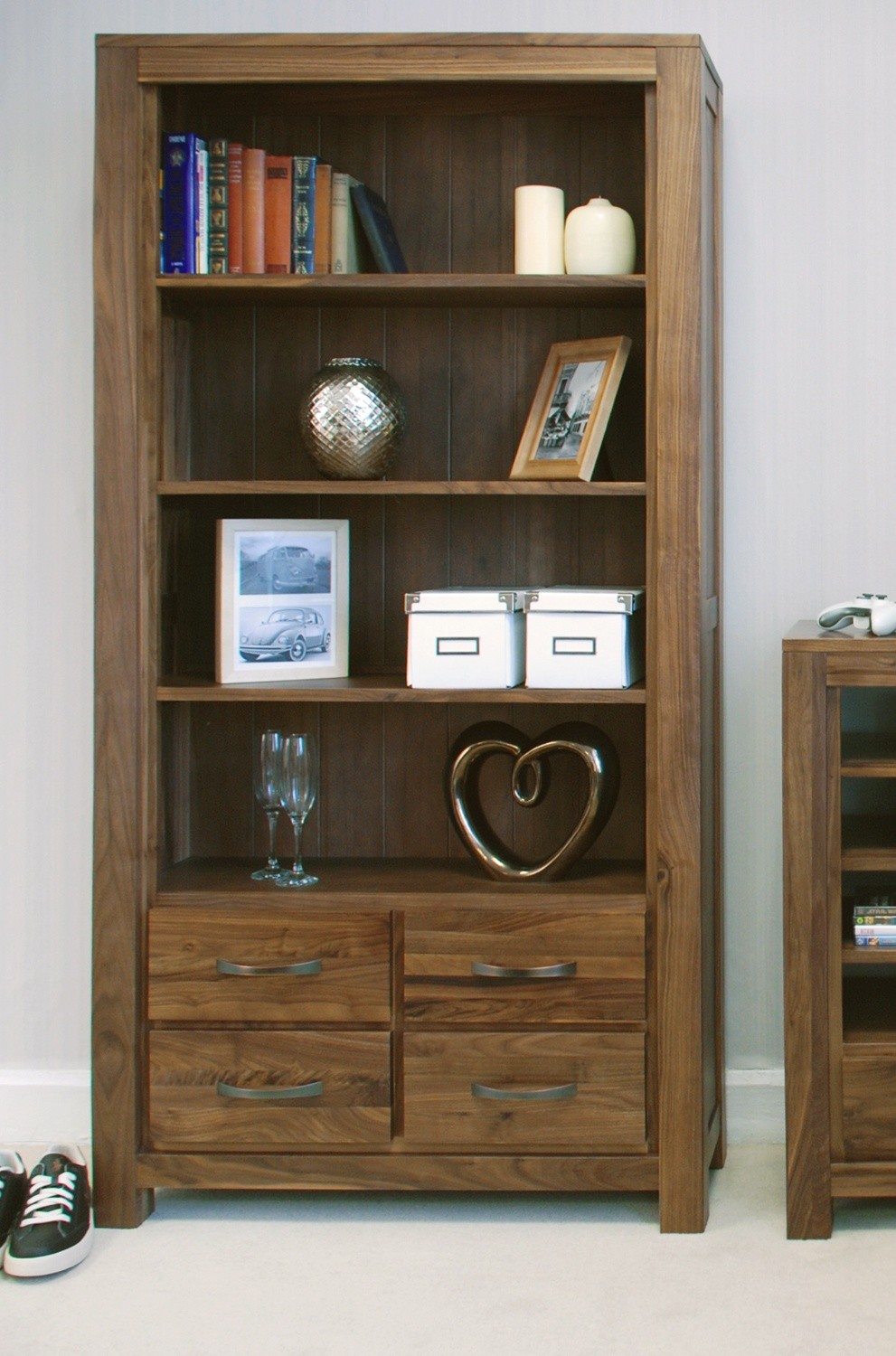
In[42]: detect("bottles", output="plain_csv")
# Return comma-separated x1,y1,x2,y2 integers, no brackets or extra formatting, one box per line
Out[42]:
297,354,408,481
565,197,636,278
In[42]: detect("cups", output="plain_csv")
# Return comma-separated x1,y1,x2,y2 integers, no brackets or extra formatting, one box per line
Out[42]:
513,184,565,273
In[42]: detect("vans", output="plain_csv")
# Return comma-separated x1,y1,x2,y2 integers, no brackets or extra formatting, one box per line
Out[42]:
254,546,321,594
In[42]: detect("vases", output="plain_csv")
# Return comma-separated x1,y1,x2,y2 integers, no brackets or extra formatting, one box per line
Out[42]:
295,356,408,478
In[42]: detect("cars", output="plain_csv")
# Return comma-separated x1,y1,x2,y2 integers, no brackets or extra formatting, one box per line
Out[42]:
238,607,331,662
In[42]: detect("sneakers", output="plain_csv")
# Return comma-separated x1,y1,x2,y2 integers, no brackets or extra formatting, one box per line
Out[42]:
6,1142,107,1277
0,1149,32,1271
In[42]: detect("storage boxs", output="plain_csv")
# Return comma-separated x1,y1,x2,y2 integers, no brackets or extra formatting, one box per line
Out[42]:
524,584,647,689
405,585,544,688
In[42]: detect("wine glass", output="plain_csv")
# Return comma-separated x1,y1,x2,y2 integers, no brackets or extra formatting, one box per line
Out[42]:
276,733,320,888
250,728,292,882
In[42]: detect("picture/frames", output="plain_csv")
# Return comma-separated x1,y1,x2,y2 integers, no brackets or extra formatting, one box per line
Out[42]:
216,515,352,686
510,335,634,481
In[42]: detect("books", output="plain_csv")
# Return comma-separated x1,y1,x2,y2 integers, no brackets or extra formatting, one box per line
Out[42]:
315,165,341,273
852,884,896,946
292,157,322,274
197,138,292,275
351,183,408,273
332,172,365,274
159,133,206,275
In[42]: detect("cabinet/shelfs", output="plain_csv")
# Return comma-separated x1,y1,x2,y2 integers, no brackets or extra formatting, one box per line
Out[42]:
781,619,896,1241
93,30,729,1233
